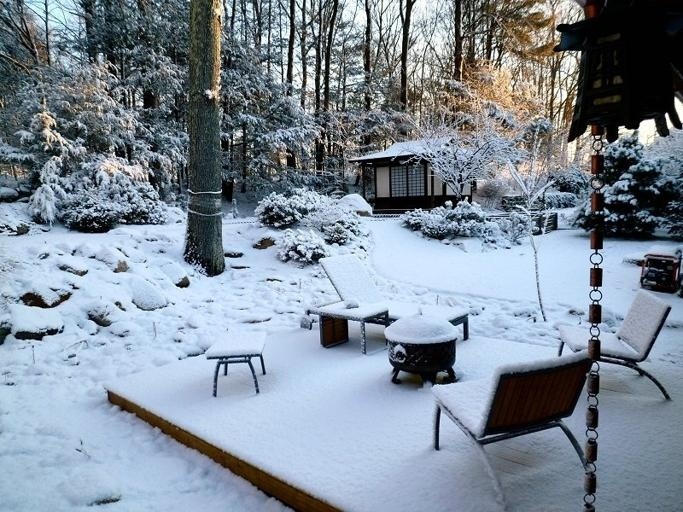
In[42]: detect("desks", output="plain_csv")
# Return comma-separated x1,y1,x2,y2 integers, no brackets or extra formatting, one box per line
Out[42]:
307,296,392,356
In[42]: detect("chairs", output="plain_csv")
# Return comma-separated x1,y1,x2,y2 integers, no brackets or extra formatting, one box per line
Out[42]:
429,349,591,512
555,289,673,403
317,252,473,341
623,246,683,298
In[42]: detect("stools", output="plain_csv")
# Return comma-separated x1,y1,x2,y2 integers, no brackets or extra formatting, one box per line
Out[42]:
205,329,268,399
383,326,459,387
